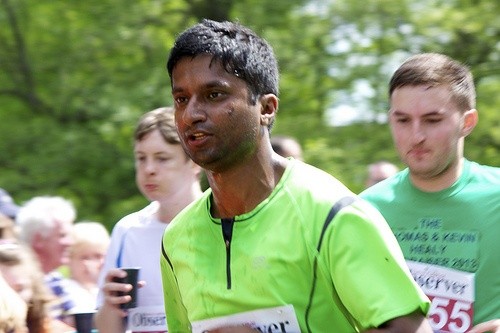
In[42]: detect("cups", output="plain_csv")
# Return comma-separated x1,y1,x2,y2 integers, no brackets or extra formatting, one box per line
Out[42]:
114,268,140,309
71,312,95,333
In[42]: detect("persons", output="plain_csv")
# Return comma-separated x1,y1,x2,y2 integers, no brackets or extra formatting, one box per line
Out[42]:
93,106,202,333
161,21,430,333
0,138,399,333
357,53,500,333
